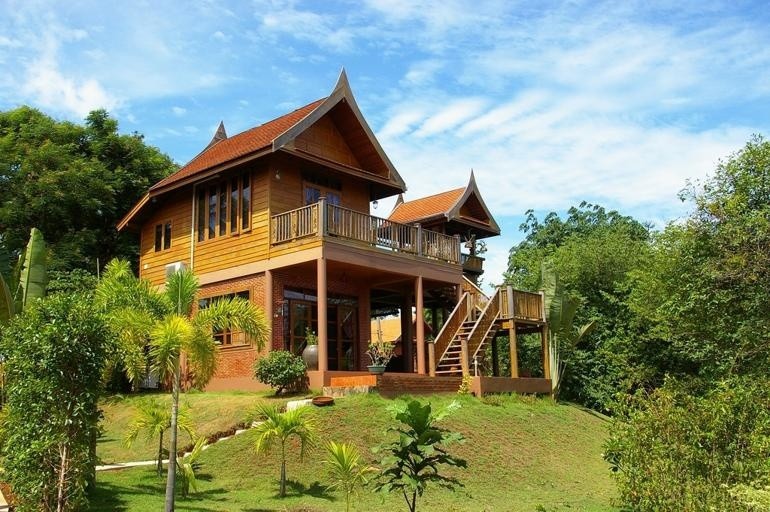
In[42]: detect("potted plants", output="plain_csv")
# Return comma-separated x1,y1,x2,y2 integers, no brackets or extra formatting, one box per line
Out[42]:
365,339,398,375
302,327,318,371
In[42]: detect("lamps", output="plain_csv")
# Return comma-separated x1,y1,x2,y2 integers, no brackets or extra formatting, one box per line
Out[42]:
372,200,378,209
272,167,280,180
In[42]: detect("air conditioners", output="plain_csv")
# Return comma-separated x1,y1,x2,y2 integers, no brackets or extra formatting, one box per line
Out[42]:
165,261,186,276
137,366,160,389
